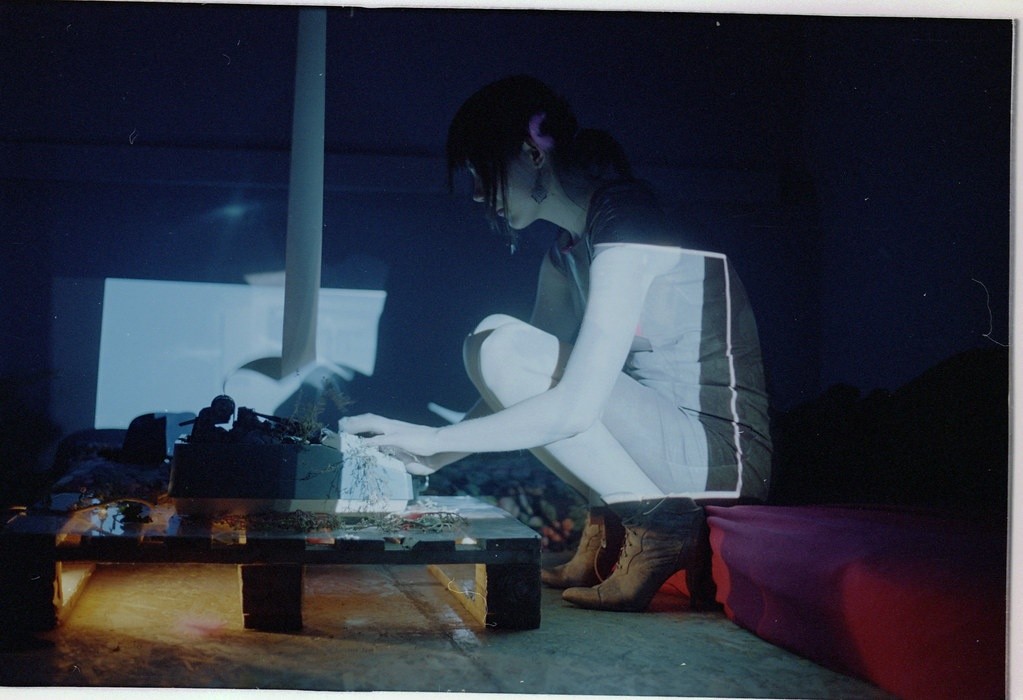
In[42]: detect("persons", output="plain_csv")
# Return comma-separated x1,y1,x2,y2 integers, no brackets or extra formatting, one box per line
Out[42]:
338,73,773,613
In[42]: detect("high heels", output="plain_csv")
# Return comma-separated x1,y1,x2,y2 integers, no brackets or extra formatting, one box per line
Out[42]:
561,506,712,613
538,504,624,589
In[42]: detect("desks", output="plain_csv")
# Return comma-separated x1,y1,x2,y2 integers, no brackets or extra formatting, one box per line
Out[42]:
0,495,542,633
